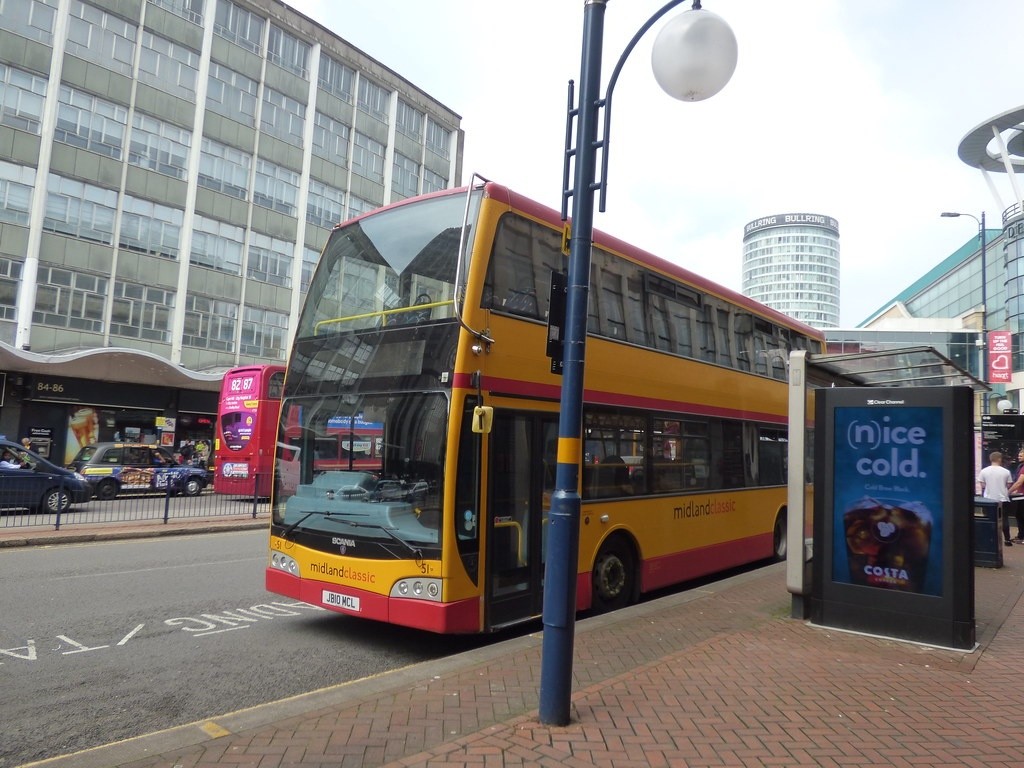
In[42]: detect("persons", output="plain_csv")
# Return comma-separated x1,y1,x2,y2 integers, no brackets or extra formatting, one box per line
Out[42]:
21,438,40,455
152,450,166,465
1007,450,1024,544
179,438,209,469
980,452,1013,546
0,450,26,470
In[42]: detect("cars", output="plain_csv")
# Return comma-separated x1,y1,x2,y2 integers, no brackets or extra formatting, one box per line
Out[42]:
0,440,92,514
66,442,211,500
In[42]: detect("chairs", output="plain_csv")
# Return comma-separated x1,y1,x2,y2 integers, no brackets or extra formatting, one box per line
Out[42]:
583,448,678,496
401,294,433,323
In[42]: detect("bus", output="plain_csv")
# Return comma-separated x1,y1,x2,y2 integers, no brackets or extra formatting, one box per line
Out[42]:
266,173,828,634
214,363,385,497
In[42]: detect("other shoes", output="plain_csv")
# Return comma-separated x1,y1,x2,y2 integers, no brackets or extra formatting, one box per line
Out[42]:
1014,537,1024,545
1004,540,1013,546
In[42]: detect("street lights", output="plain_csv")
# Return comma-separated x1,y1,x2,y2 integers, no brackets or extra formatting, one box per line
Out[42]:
940,211,987,414
539,0,739,726
980,392,1012,414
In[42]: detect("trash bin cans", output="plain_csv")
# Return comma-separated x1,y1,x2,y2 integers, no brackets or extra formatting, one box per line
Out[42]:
974,496,1003,567
